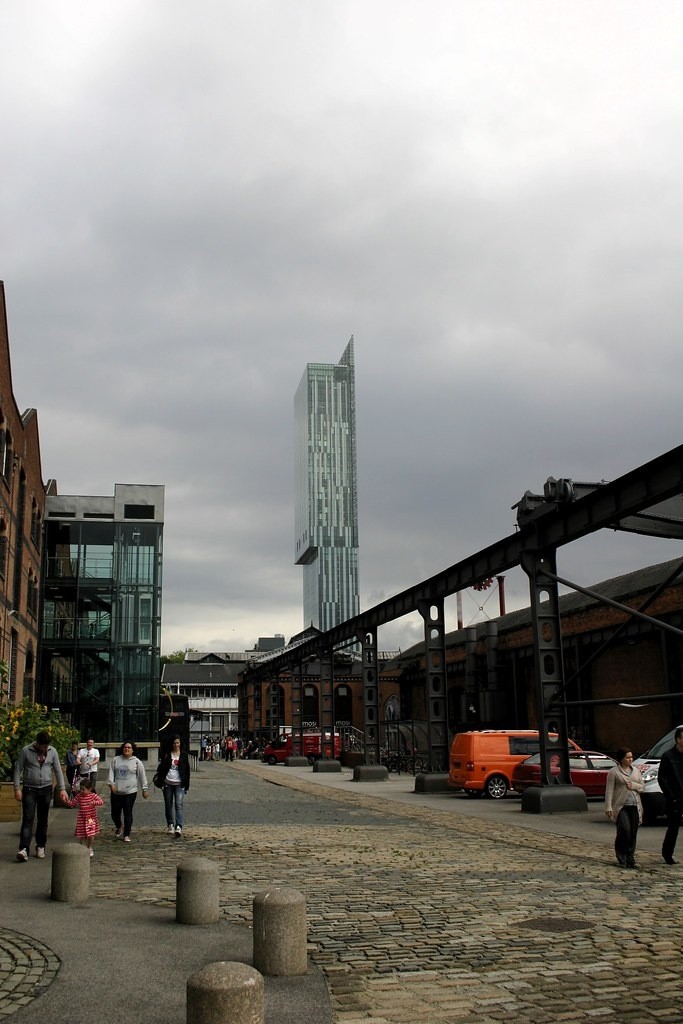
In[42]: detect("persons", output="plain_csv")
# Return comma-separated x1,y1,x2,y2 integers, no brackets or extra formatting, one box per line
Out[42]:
199,732,268,762
66,737,100,801
657,725,683,864
106,740,149,842
63,778,104,859
604,748,645,870
157,736,190,838
13,732,70,861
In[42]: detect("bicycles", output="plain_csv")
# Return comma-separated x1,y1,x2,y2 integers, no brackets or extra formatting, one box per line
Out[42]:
383,748,441,775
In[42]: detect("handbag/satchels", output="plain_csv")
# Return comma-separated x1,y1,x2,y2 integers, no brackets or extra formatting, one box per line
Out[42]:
152,773,164,788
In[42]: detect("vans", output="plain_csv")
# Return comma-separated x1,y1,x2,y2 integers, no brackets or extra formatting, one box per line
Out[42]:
446,727,584,799
629,724,683,825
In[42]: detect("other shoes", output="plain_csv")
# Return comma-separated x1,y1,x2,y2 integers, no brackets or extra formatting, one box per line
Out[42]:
662,853,674,864
115,825,123,837
89,848,94,857
629,862,642,870
124,836,131,842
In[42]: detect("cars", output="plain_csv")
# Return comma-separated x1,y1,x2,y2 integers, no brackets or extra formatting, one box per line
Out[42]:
510,748,620,798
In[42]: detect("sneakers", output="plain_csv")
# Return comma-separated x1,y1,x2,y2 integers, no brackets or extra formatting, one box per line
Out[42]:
36,847,45,859
175,825,182,837
167,825,174,834
16,848,28,862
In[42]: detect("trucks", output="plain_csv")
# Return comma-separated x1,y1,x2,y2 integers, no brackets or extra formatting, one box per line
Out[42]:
260,730,342,766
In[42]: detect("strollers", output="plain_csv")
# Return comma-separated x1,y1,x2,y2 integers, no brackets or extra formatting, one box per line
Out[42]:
67,764,93,809
